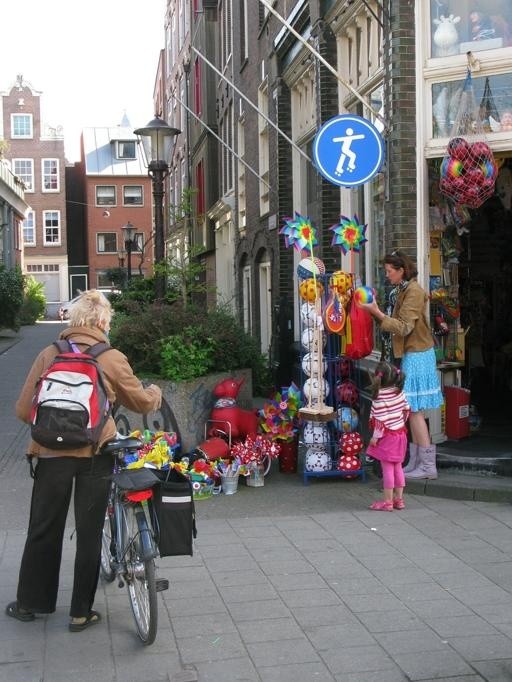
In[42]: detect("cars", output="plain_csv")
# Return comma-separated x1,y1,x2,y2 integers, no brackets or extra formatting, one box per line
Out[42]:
59,289,122,320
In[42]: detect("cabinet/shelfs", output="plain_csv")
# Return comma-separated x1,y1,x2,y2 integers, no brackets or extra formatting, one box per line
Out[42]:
431,204,492,390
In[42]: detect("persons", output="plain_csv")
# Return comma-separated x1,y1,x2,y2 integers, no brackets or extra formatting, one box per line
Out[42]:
366,363,410,512
356,250,445,480
6,291,162,632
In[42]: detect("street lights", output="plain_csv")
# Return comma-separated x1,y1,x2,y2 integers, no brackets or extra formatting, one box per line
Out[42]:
133,110,182,302
121,221,138,280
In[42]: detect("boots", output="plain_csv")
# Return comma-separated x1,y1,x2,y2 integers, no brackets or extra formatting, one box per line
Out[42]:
405,445,438,480
403,442,418,472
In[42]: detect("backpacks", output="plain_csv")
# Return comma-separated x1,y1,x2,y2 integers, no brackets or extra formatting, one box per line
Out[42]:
341,292,374,360
30,340,115,457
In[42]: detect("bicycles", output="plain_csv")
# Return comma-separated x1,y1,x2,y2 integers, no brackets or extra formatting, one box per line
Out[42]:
99,432,170,647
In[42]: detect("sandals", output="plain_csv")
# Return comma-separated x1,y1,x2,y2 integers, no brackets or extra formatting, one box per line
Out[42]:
393,497,405,509
368,499,393,512
68,610,101,633
5,600,35,622
